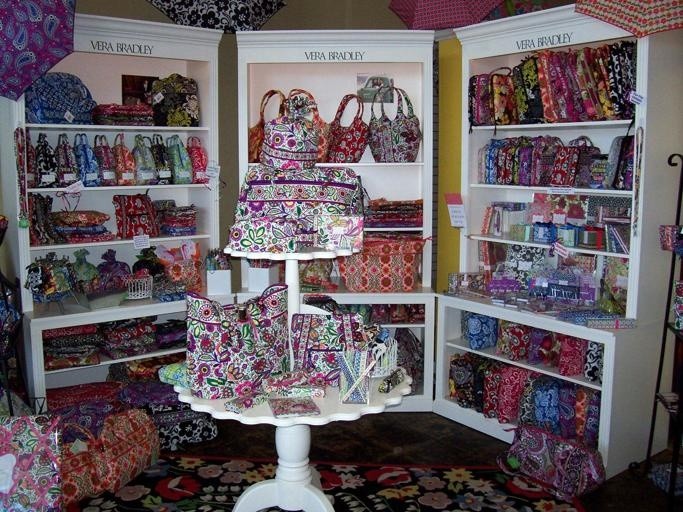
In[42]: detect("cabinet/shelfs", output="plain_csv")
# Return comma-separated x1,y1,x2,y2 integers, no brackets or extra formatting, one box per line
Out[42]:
234,27,435,415
433,3,682,482
155,241,416,512
0,3,228,417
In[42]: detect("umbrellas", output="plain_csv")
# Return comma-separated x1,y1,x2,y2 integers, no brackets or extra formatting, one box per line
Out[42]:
573,0,682,38
387,0,505,29
147,1,288,34
1,0,77,102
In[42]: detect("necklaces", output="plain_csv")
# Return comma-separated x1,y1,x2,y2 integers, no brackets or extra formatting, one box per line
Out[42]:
14,127,29,228
632,126,643,236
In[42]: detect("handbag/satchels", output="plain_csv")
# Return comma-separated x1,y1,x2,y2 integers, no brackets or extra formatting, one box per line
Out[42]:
186,284,367,402
27,132,209,188
449,40,637,452
227,82,422,293
497,425,605,502
25,194,197,247
25,71,201,127
